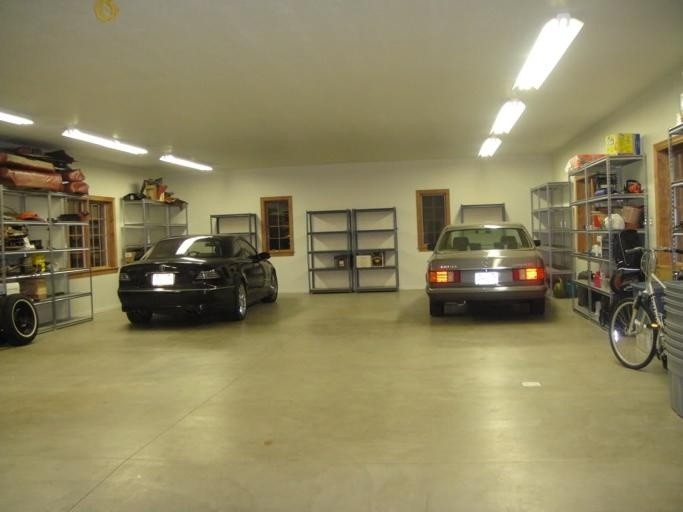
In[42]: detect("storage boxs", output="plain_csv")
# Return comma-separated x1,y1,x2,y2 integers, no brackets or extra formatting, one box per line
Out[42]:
18,278,47,300
145,184,165,202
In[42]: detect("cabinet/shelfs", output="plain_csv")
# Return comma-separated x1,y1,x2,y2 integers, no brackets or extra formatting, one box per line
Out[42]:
305,206,399,294
210,213,258,251
120,197,187,267
0,183,94,335
530,123,683,335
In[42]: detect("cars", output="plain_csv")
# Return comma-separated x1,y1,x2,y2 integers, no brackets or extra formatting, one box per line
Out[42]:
117,234,278,324
425,223,549,316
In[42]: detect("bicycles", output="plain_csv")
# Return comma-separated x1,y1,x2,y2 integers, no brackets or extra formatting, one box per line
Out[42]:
608,246,665,370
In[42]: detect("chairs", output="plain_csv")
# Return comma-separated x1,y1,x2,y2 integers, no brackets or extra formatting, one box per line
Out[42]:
494,236,518,248
452,237,481,251
